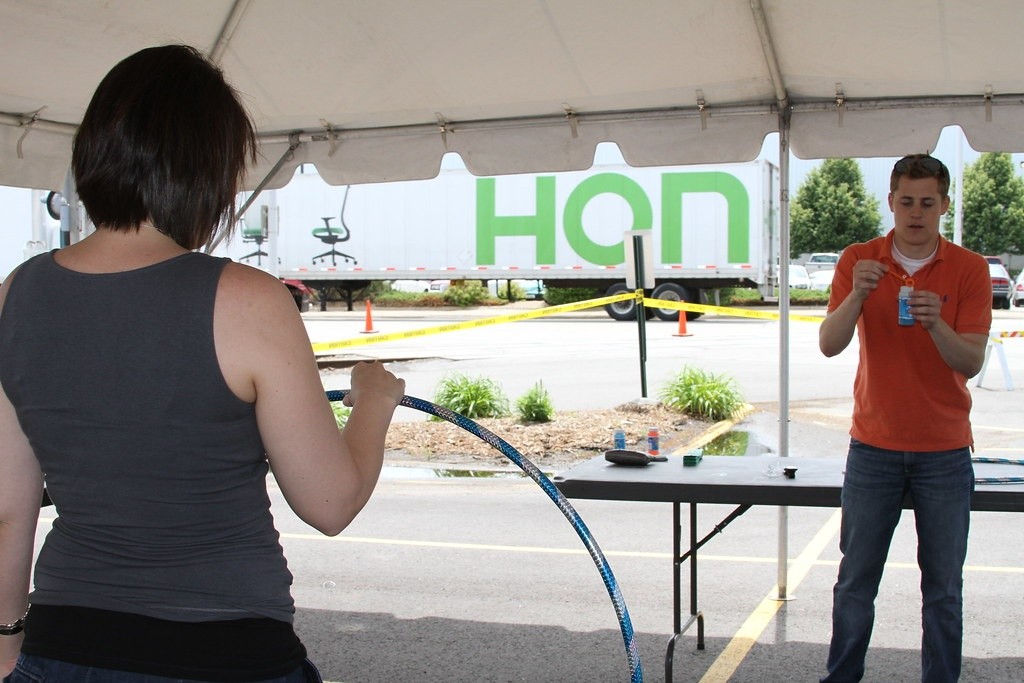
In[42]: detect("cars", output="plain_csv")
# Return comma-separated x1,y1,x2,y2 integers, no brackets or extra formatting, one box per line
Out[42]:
988,263,1010,309
1013,268,1024,307
776,265,811,289
984,255,1007,268
809,270,834,291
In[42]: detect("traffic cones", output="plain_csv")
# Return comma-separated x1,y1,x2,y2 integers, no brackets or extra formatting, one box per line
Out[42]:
360,298,379,333
672,301,693,337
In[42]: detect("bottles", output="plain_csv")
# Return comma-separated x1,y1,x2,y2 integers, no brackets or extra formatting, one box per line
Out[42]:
898,286,914,326
613,429,625,449
647,426,659,455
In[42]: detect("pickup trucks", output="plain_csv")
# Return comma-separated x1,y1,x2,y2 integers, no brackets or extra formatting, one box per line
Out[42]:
805,252,840,274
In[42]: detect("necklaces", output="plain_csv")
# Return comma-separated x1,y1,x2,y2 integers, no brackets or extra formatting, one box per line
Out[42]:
139,221,160,230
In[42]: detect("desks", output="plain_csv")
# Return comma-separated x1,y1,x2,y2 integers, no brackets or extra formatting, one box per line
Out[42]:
550,448,1024,683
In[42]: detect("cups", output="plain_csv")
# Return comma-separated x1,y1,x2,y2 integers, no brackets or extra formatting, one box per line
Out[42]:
761,453,779,475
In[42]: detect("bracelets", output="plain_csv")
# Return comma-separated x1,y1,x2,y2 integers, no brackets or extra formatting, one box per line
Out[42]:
0,603,31,635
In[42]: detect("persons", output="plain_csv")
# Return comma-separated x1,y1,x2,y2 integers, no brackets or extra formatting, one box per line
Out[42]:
820,155,993,683
0,44,404,683
